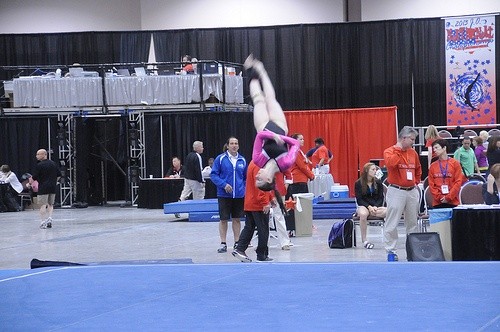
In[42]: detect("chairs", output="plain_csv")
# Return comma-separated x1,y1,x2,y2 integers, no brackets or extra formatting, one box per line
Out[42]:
352,173,486,247
438,130,452,138
488,129,500,135
464,130,477,136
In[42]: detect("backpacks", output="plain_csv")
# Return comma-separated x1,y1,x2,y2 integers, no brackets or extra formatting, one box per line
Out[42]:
327,217,357,248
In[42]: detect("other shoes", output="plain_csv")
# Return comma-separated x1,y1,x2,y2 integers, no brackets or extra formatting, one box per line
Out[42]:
40,221,47,229
234,240,254,249
386,253,399,261
363,242,375,249
256,256,274,262
231,249,252,263
217,244,227,253
281,242,296,250
46,217,52,228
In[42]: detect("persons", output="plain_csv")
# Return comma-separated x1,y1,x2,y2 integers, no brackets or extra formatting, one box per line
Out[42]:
180,55,200,75
353,122,500,262
243,54,301,192
210,136,248,251
163,133,333,261
0,164,39,212
32,148,61,230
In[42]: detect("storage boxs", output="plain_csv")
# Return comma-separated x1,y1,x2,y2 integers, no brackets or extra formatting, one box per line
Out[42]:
330,185,350,200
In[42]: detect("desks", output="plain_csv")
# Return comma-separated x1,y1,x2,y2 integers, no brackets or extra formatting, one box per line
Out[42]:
451,204,500,262
138,177,217,209
13,74,244,107
308,174,334,200
444,136,491,153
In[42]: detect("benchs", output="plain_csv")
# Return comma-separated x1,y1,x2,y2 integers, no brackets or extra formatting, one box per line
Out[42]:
18,193,33,209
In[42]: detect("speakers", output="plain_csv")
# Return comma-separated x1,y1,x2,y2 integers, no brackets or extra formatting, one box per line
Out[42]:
405,232,446,261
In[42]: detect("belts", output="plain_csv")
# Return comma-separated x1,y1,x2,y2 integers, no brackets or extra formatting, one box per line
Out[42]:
390,184,416,190
